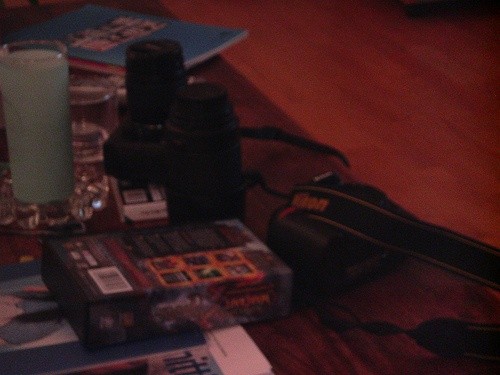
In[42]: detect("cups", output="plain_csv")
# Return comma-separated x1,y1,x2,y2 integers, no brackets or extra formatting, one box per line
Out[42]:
1,39,77,226
64,71,117,209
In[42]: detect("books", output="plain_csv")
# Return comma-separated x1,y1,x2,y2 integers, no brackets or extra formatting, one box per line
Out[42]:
7,1,249,81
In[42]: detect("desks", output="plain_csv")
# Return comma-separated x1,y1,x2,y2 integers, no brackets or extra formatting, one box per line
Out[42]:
0,0,500,375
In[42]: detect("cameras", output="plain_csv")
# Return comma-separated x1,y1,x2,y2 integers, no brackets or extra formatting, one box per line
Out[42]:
103,40,243,213
266,181,412,293
